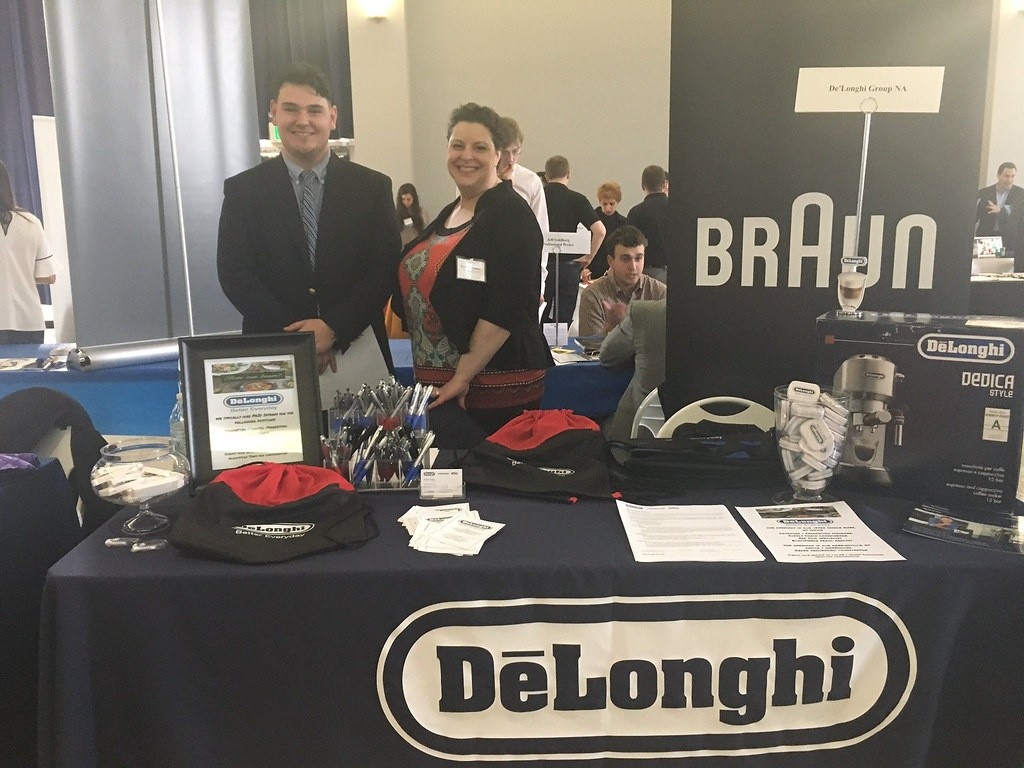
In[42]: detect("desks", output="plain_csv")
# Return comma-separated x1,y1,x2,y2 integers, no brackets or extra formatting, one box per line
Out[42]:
37,489,1024,768
969,272,1024,318
385,338,635,460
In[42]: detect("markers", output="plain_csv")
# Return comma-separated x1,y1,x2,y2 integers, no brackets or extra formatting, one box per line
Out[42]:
43,357,57,370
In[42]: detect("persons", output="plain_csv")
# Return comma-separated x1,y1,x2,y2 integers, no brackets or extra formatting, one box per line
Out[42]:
578,225,667,339
218,61,401,439
393,102,553,451
536,154,605,332
0,160,56,345
627,165,670,286
456,118,550,313
598,299,667,453
580,181,628,282
975,162,1024,274
395,182,428,251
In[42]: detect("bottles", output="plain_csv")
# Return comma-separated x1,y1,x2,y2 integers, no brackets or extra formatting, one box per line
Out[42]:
268,111,283,144
169,393,185,456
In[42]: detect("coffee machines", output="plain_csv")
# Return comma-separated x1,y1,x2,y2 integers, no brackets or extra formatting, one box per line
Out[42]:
831,353,905,485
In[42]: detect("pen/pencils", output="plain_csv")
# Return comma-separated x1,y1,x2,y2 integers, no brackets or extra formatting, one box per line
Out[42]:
320,424,436,487
329,373,433,432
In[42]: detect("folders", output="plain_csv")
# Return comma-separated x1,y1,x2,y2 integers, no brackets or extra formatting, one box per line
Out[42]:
573,337,603,352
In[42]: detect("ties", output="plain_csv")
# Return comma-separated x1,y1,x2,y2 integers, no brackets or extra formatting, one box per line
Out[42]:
299,170,321,319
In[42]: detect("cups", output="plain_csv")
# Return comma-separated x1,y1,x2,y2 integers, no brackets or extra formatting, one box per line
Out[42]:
836,271,867,314
772,383,852,505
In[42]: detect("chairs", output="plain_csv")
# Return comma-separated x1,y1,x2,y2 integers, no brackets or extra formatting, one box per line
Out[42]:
656,396,777,439
631,381,668,439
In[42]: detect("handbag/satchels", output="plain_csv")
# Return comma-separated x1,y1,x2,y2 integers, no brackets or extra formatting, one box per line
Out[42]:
166,460,379,563
451,404,625,504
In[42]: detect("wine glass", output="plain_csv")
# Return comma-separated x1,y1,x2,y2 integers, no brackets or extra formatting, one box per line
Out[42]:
91,437,191,536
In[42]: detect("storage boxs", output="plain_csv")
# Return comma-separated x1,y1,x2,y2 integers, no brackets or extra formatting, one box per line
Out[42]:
973,236,1003,260
814,310,1024,514
